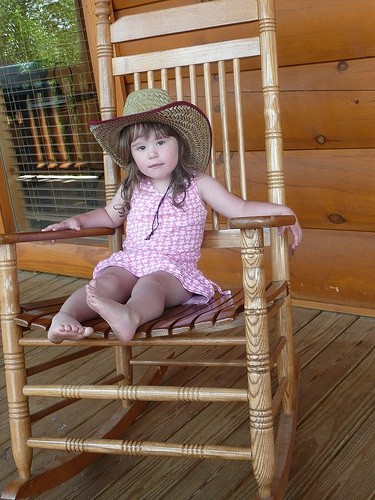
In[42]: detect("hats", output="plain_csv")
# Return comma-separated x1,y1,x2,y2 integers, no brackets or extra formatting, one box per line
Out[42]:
90,89,213,174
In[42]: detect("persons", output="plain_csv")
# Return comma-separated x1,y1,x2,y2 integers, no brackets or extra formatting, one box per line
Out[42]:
40,88,302,345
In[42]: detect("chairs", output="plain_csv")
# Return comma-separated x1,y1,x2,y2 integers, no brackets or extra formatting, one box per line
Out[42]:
0,0,300,500
